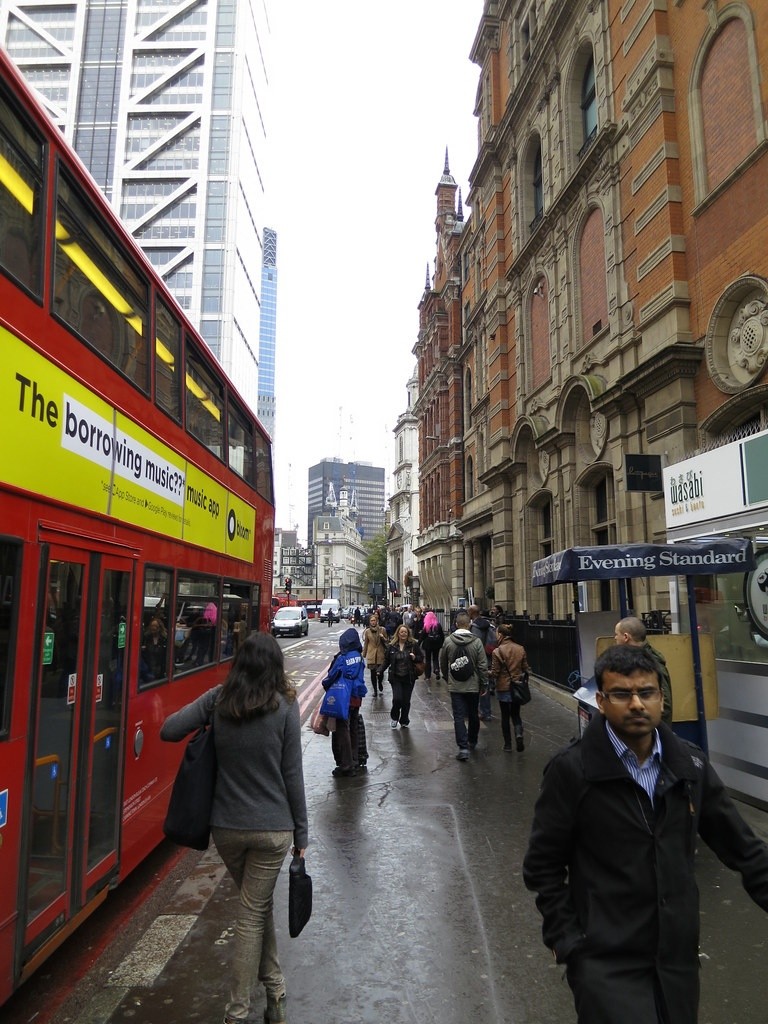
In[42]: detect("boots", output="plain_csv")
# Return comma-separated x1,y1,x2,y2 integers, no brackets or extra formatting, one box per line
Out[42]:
514,724,525,752
372,679,377,697
501,731,512,751
378,677,384,692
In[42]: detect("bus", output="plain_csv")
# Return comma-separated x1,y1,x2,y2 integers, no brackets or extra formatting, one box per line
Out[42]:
272,592,297,617
0,52,276,1003
297,599,322,618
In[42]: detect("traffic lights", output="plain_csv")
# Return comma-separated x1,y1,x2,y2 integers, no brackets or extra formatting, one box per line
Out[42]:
285,578,291,594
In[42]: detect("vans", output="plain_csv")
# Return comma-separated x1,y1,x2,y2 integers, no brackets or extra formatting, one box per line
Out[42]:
320,599,341,623
273,606,309,638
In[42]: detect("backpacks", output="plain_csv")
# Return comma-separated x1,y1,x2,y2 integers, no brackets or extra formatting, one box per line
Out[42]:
450,644,475,682
427,628,440,647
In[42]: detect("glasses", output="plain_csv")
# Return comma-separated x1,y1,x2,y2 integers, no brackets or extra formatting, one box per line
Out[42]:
600,687,665,704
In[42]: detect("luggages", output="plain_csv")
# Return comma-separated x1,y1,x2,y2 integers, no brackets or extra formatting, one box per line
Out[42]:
358,714,369,766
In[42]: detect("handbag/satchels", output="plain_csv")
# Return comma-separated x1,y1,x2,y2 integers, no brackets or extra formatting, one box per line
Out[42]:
411,641,426,677
381,639,390,659
163,684,224,851
408,613,416,629
484,627,498,657
509,672,533,706
289,849,313,939
310,654,353,737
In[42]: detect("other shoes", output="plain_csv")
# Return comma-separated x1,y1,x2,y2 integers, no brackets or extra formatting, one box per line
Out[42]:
391,721,408,728
478,712,495,721
269,992,286,1002
332,766,361,778
424,669,440,681
455,750,469,760
222,1018,240,1024
467,742,476,750
489,688,495,697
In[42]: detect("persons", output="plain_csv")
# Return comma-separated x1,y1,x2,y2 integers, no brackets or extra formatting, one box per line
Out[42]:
615,616,673,730
141,604,533,776
160,630,310,1024
522,642,768,1024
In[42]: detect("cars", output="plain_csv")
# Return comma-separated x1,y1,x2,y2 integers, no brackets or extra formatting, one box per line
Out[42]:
341,604,384,624
